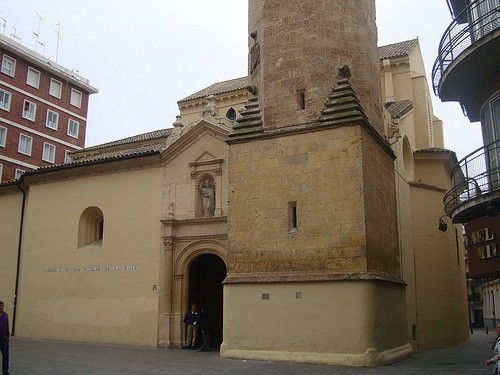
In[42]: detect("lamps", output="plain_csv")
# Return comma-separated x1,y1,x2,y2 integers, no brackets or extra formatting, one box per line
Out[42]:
439,214,451,232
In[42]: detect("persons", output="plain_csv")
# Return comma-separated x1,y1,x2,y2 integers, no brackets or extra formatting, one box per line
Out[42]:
485,322,500,375
180,303,200,349
198,179,216,217
